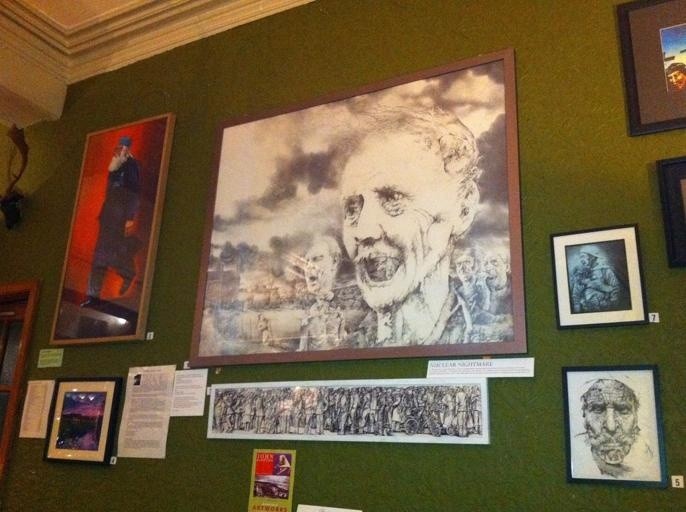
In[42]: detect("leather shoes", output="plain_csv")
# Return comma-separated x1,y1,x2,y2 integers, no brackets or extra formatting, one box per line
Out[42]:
79,298,102,309
118,272,138,295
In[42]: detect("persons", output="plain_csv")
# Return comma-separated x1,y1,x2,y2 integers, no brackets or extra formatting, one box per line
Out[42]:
211,383,483,439
573,371,660,482
666,62,686,93
572,264,611,312
568,244,628,312
274,454,290,476
292,101,516,353
79,135,142,308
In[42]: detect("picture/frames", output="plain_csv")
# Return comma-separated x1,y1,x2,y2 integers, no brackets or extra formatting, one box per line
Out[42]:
186,45,530,370
616,2,685,136
654,153,683,271
550,221,647,329
43,377,125,468
562,364,666,487
49,111,178,345
207,377,490,445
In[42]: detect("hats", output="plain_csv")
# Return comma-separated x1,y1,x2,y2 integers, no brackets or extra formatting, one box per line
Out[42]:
117,135,131,147
